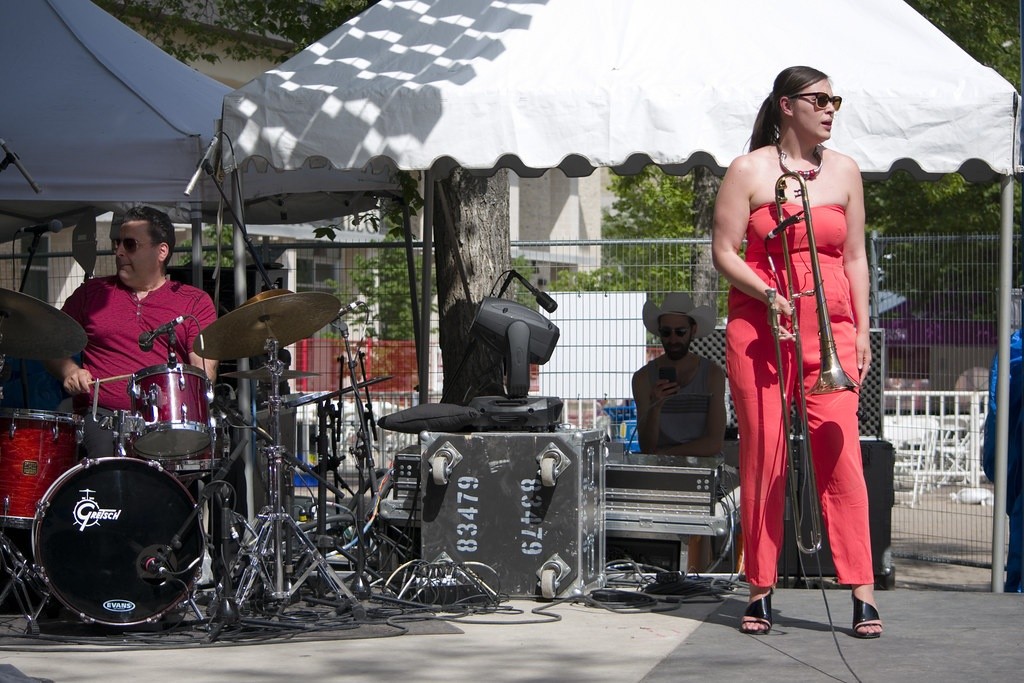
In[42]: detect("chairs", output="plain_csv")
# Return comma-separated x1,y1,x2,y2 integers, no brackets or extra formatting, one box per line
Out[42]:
878,413,987,512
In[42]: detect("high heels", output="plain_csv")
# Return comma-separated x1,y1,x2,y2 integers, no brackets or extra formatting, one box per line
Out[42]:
740,585,772,633
852,590,882,638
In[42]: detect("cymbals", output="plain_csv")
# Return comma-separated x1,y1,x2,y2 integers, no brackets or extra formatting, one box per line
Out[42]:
192,289,343,361
0,284,88,361
219,366,326,384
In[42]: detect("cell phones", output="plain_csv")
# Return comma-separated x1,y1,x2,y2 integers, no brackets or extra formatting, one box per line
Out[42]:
658,367,678,394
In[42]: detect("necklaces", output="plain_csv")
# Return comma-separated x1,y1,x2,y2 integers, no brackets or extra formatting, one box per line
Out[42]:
776,136,823,180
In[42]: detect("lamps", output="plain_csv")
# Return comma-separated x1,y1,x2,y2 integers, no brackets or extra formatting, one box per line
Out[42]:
470,295,563,432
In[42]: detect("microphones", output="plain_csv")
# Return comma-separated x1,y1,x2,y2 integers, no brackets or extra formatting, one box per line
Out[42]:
184,131,222,196
338,301,362,317
0,138,42,193
210,402,231,424
19,220,64,234
140,556,167,575
137,315,190,351
764,211,802,242
515,272,558,313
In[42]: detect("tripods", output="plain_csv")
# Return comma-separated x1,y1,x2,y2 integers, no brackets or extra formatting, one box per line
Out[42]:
163,319,442,644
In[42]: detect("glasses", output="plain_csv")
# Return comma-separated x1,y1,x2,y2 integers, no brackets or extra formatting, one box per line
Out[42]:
788,92,842,112
658,323,692,337
112,237,168,253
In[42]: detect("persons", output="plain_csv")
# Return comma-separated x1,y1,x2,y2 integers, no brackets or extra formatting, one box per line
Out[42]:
711,66,883,639
632,293,727,457
58,207,271,590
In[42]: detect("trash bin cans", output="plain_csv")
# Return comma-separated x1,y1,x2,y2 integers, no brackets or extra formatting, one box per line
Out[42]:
603,406,642,453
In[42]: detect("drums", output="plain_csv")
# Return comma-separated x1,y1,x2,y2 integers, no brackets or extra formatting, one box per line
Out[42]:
158,416,225,472
30,453,207,629
126,362,214,461
0,406,86,530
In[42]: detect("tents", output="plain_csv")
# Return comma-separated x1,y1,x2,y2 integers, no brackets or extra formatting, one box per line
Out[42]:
0,0,424,410
222,0,1021,595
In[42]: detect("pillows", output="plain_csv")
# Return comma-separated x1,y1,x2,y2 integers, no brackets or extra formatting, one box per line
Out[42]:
376,401,480,433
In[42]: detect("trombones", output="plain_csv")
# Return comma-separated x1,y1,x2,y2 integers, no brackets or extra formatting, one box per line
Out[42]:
762,169,863,559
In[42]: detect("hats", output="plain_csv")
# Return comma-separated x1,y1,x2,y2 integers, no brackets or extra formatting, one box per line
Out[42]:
642,293,717,338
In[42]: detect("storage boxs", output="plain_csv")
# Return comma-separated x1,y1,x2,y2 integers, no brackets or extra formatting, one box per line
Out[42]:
415,425,606,598
